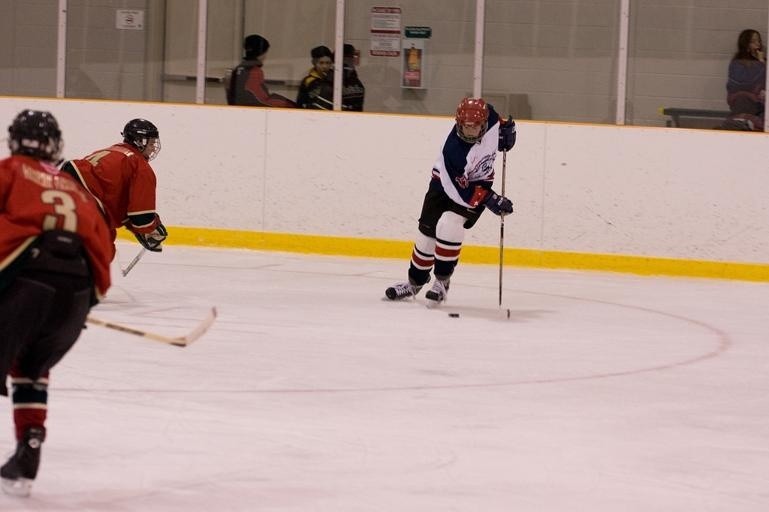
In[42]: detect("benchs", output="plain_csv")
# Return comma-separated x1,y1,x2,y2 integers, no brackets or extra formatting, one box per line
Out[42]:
660,107,735,128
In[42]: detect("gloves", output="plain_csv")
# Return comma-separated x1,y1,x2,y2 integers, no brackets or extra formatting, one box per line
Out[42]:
497,115,518,153
134,221,169,254
481,189,515,218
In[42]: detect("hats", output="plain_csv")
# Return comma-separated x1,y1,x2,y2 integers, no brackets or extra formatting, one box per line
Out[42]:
310,45,332,68
244,34,269,60
333,43,357,63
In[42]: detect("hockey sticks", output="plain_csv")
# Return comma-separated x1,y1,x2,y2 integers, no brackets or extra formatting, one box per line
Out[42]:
84,307,218,347
499,147,510,319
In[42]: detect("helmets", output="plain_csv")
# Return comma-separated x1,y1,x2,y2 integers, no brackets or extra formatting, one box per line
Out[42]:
455,96,489,143
6,109,63,163
121,117,159,153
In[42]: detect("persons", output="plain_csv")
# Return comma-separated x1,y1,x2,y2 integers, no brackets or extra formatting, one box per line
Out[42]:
310,44,364,111
61,117,167,262
385,96,517,304
226,34,296,108
297,45,331,108
726,29,766,118
0,109,115,484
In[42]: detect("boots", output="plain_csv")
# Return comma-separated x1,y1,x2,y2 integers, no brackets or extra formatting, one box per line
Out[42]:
0,425,47,482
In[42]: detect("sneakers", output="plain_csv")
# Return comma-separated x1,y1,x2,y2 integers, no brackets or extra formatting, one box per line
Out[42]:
425,276,453,300
385,280,423,300
